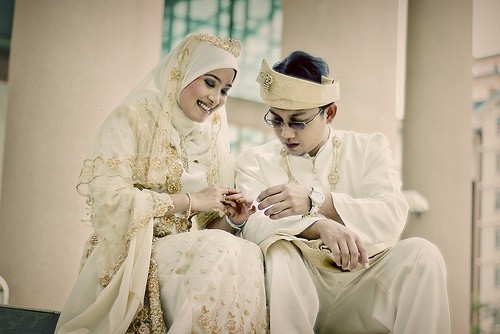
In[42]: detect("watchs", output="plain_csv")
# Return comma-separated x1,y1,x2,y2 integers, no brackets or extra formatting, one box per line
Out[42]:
301,186,326,218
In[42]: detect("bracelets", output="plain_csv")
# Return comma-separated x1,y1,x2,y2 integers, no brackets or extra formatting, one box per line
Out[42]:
185,192,193,219
225,214,248,230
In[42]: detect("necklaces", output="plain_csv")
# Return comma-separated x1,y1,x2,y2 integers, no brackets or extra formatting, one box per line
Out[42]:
280,135,342,192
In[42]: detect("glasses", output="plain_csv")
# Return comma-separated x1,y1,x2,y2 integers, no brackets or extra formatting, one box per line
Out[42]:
263,109,324,131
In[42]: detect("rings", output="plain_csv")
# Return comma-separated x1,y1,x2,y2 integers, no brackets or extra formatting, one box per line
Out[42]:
223,194,226,203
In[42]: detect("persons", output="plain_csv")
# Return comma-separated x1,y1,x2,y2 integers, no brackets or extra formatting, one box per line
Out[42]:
232,51,452,334
56,32,268,334
